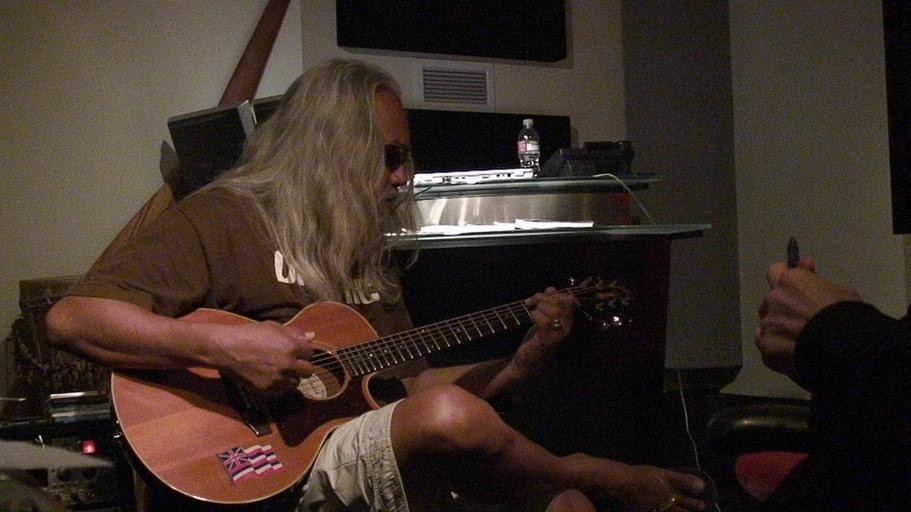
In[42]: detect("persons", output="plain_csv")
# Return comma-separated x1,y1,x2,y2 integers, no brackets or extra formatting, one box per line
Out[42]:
754,254,911,510
46,60,708,511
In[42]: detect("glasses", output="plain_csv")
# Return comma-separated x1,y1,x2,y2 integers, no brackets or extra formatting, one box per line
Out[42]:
382,144,414,167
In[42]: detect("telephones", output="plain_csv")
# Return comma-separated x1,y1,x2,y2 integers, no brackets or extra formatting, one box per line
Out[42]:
543,140,635,177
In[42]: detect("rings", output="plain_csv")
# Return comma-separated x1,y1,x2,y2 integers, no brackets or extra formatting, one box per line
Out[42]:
551,316,565,331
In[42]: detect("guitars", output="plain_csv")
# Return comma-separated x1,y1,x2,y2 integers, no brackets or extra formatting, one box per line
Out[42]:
109,275,640,506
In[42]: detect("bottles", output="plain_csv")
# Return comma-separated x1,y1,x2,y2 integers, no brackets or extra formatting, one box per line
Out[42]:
517,118,543,176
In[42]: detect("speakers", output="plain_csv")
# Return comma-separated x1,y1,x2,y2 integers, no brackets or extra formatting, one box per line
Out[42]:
168,98,254,194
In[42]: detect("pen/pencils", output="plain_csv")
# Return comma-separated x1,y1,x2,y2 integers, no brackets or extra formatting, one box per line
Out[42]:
787,237,799,269
